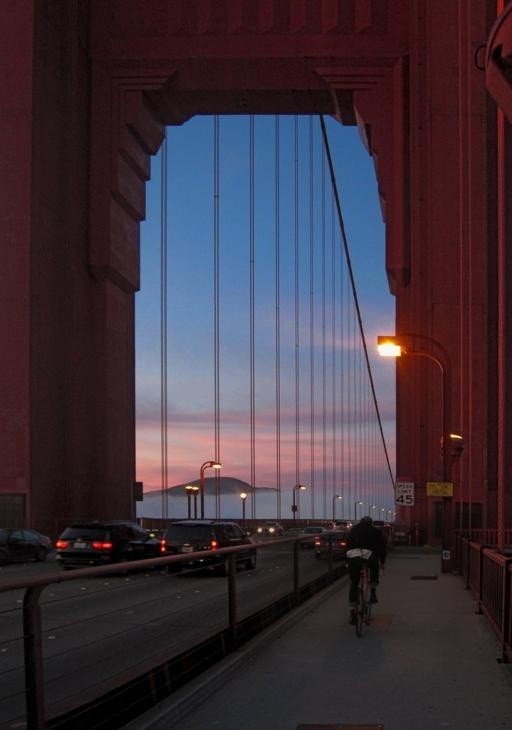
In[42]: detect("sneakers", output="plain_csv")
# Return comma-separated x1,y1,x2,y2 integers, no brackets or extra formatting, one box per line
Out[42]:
368,588,378,604
348,608,358,625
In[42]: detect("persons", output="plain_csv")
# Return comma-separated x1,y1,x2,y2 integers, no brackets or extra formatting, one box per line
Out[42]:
343,515,386,620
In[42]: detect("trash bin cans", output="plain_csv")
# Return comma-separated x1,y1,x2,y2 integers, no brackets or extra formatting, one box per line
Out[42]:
440,550,453,574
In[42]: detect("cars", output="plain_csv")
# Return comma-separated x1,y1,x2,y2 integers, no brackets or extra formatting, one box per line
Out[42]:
315,529,354,561
1,528,53,566
258,519,284,538
328,519,393,533
297,526,327,550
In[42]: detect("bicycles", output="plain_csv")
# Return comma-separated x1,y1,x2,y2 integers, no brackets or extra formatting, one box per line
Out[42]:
347,559,380,639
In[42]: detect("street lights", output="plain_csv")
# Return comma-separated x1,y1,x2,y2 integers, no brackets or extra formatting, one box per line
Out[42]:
185,486,199,520
332,495,341,526
200,461,221,520
240,493,247,527
376,335,458,574
353,501,396,523
292,485,306,528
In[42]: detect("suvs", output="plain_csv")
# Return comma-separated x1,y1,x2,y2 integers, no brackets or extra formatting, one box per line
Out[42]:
55,518,162,572
161,518,256,575
